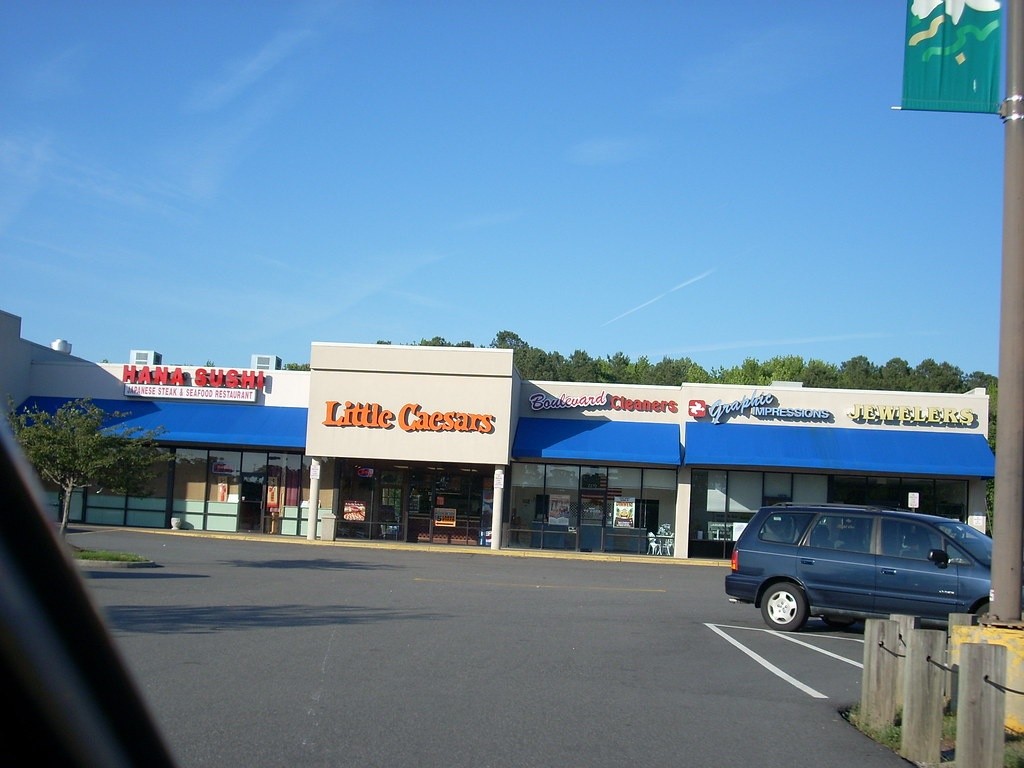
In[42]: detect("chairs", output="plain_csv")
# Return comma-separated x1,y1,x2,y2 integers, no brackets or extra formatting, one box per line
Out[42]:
645,532,662,555
664,533,674,555
815,524,954,566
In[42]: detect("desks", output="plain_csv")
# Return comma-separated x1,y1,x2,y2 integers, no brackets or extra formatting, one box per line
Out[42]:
656,535,674,555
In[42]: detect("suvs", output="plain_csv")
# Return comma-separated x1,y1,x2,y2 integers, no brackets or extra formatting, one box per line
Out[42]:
723,500,1024,632
